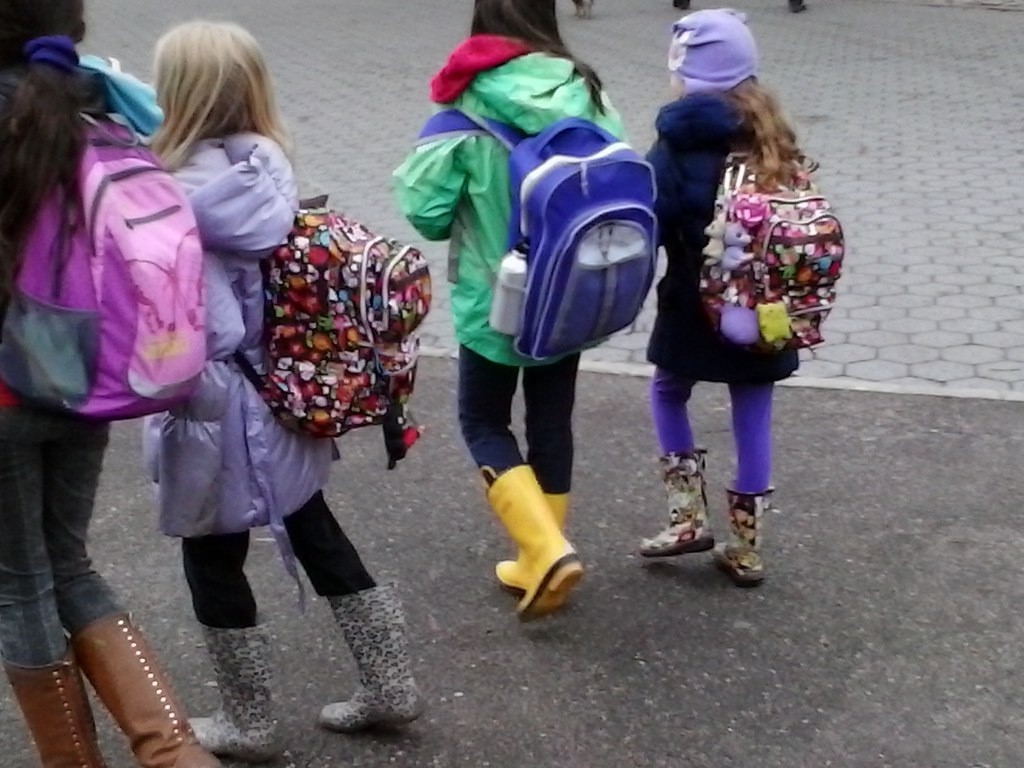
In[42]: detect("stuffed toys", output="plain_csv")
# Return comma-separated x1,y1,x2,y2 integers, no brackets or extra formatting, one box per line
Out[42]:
704,210,755,283
721,302,792,344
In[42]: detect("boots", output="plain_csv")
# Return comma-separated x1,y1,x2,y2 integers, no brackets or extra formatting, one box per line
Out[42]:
2,628,106,768
486,463,584,619
495,488,570,595
69,612,223,768
712,481,775,587
640,448,716,558
188,620,288,763
319,581,423,730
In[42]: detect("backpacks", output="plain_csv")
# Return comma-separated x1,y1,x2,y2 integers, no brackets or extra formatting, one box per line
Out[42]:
229,193,433,467
700,149,846,349
0,110,207,422
419,106,661,359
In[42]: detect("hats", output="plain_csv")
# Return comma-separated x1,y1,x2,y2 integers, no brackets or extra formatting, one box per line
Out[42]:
667,7,760,100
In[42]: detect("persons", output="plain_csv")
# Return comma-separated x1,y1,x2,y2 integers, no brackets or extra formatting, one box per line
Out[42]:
142,21,434,763
0,0,226,768
393,0,628,621
637,8,806,580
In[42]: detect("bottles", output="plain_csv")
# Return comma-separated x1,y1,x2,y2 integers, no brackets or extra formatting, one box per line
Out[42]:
490,236,532,338
